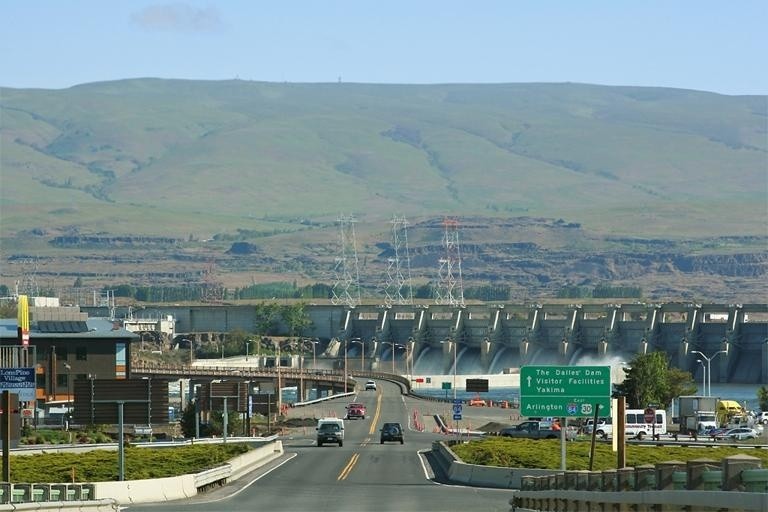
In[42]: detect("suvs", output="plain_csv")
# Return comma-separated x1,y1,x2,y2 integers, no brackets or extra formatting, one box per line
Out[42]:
380,422,404,445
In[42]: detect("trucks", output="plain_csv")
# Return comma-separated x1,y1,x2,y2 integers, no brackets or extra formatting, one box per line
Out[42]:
679,395,743,434
315,417,345,447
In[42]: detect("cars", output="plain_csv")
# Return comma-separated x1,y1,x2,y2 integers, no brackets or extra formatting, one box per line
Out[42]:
345,402,366,419
711,412,768,439
366,380,377,391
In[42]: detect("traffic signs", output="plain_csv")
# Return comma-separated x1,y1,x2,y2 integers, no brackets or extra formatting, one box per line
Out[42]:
520,365,611,418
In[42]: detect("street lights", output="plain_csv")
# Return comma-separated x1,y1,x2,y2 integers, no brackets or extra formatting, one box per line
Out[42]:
395,343,414,379
343,337,365,392
690,350,726,396
278,342,297,407
440,340,457,400
182,337,193,366
245,339,260,364
382,340,395,376
301,338,317,401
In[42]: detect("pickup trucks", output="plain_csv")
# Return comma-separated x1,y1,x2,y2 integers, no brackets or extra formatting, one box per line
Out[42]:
499,421,577,440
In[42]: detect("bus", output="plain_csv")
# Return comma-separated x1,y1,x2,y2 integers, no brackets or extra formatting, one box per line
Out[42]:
584,409,667,439
